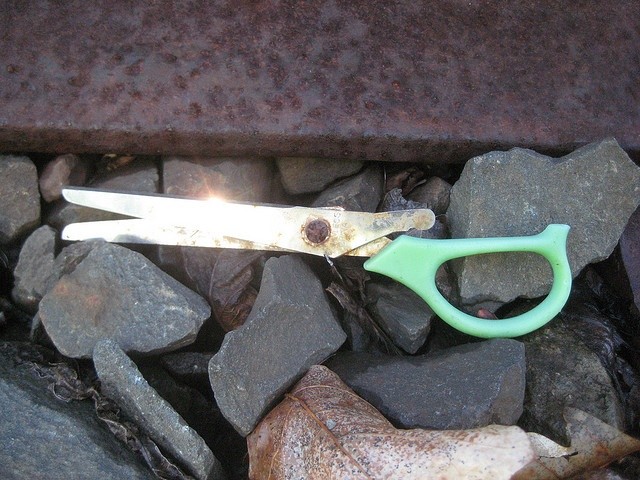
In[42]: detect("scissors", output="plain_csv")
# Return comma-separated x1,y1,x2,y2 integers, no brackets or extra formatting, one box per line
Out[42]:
60,183,573,339
